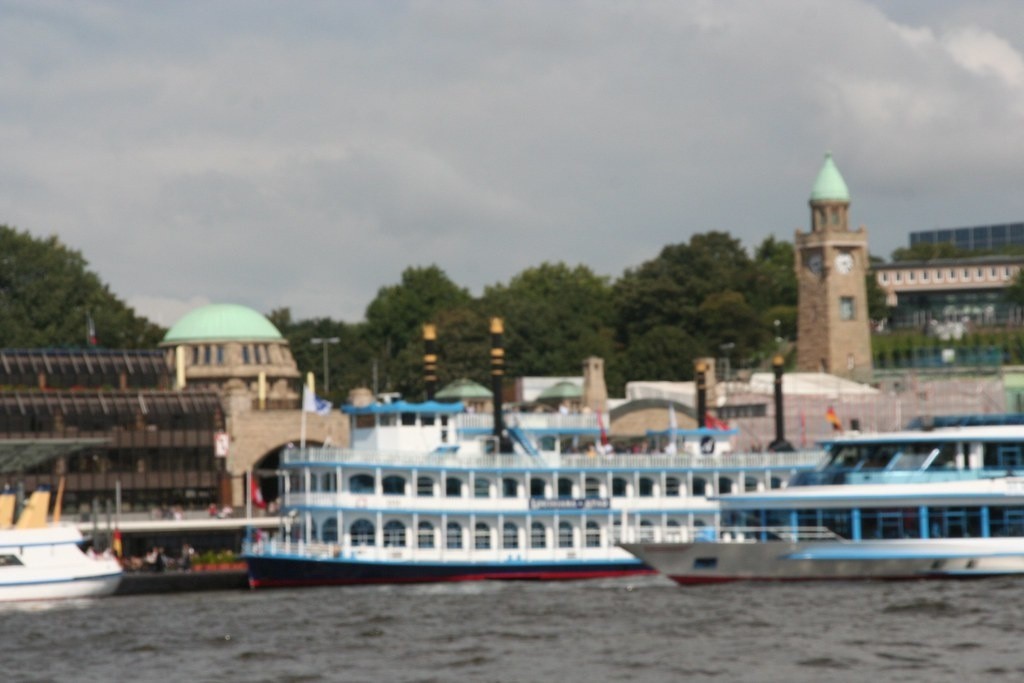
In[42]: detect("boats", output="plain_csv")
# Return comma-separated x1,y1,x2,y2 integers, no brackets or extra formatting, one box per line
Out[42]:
612,411,1024,583
1,485,122,602
239,305,837,591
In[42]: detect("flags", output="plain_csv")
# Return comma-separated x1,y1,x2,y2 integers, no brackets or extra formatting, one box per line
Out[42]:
664,400,679,457
250,476,266,509
826,405,844,436
303,386,332,417
596,408,613,454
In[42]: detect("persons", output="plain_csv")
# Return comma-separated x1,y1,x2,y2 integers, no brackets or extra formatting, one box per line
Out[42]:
561,437,664,456
465,397,580,416
79,502,90,522
87,502,284,573
1,477,15,495
